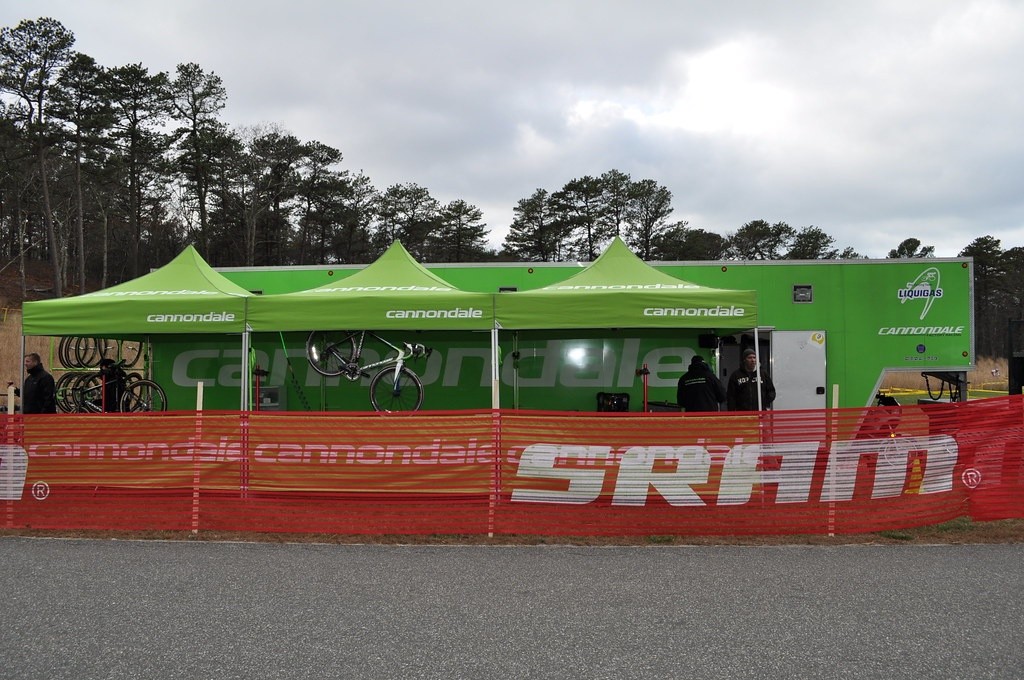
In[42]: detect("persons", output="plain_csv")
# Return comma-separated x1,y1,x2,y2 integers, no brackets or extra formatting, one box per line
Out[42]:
728,348,777,411
677,356,725,412
92,358,131,412
23,354,56,415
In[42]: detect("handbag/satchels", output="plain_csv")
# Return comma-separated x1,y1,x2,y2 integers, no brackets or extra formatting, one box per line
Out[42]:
596,392,629,412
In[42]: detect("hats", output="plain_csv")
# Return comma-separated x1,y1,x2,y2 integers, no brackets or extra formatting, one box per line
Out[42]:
743,349,755,358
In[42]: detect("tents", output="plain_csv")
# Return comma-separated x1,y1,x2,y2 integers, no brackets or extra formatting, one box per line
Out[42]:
20,237,765,505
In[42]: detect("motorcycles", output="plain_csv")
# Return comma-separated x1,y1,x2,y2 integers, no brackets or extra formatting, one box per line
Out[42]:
848,390,917,470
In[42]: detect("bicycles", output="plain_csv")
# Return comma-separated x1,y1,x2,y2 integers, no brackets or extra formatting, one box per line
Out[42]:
55,337,167,413
306,330,432,416
